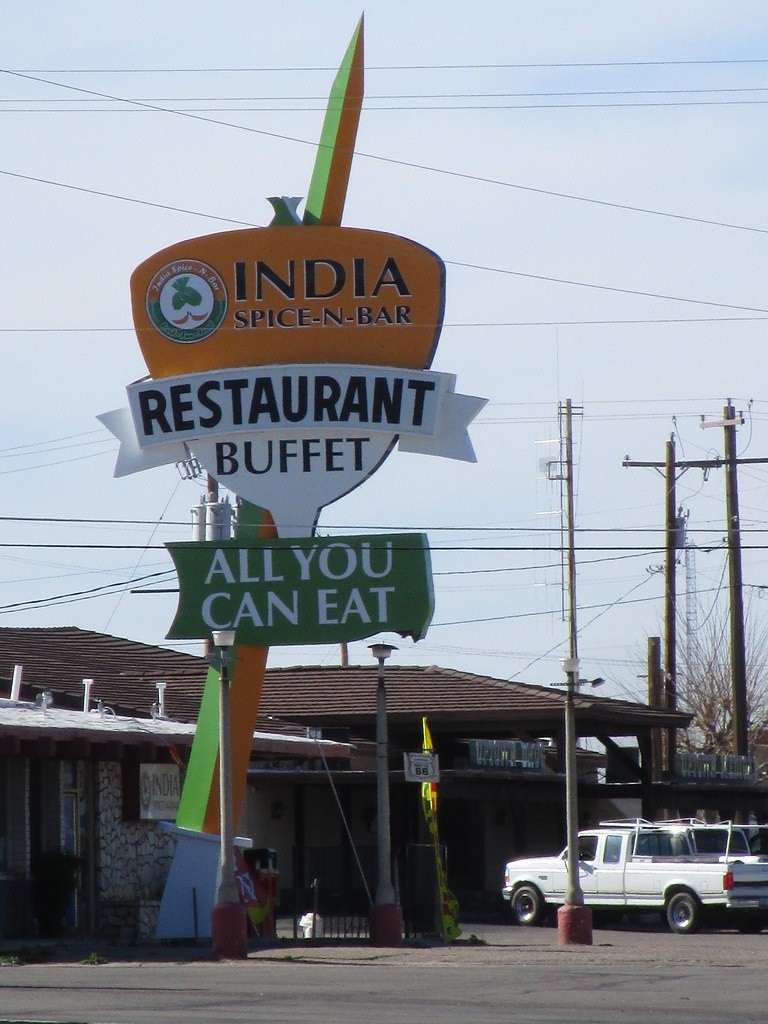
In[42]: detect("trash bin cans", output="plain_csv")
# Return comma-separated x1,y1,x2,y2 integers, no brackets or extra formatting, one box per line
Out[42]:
243,848,278,938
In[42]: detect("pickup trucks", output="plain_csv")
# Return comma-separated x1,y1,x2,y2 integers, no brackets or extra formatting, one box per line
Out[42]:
502,828,768,934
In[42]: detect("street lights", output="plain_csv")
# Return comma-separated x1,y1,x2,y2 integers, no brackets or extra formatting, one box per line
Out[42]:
212,630,248,959
558,651,593,946
367,644,404,949
